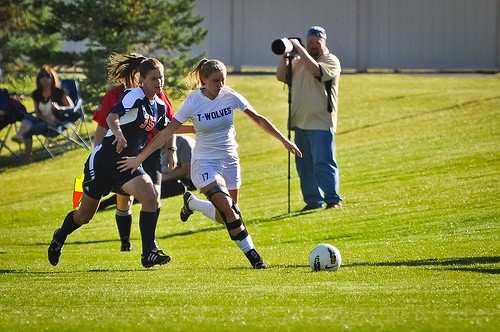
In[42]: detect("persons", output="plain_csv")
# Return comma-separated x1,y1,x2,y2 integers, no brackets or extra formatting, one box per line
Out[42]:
115,57,302,269
48,53,195,268
0,64,74,164
277,26,343,210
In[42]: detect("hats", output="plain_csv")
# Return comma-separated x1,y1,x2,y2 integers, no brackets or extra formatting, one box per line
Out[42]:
307,26,326,39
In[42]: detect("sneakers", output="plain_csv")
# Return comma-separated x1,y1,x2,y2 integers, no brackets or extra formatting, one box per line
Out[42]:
48,228,65,267
253,261,266,270
180,191,194,222
140,249,171,268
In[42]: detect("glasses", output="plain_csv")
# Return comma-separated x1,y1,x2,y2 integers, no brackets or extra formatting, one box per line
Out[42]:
39,73,53,79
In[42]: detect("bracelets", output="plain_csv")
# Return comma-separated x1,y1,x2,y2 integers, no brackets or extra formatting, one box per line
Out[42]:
167,146,178,152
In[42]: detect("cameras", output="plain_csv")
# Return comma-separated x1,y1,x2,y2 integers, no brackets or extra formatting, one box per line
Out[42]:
271,37,305,55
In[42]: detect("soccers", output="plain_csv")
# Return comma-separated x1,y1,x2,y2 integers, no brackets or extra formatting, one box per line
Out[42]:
308,244,342,271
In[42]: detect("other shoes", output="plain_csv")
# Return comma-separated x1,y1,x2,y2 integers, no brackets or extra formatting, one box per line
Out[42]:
302,206,324,211
12,135,23,143
326,203,341,209
120,246,131,251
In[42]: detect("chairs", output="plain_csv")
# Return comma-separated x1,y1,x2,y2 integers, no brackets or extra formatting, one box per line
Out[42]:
20,79,92,161
0,89,27,160
